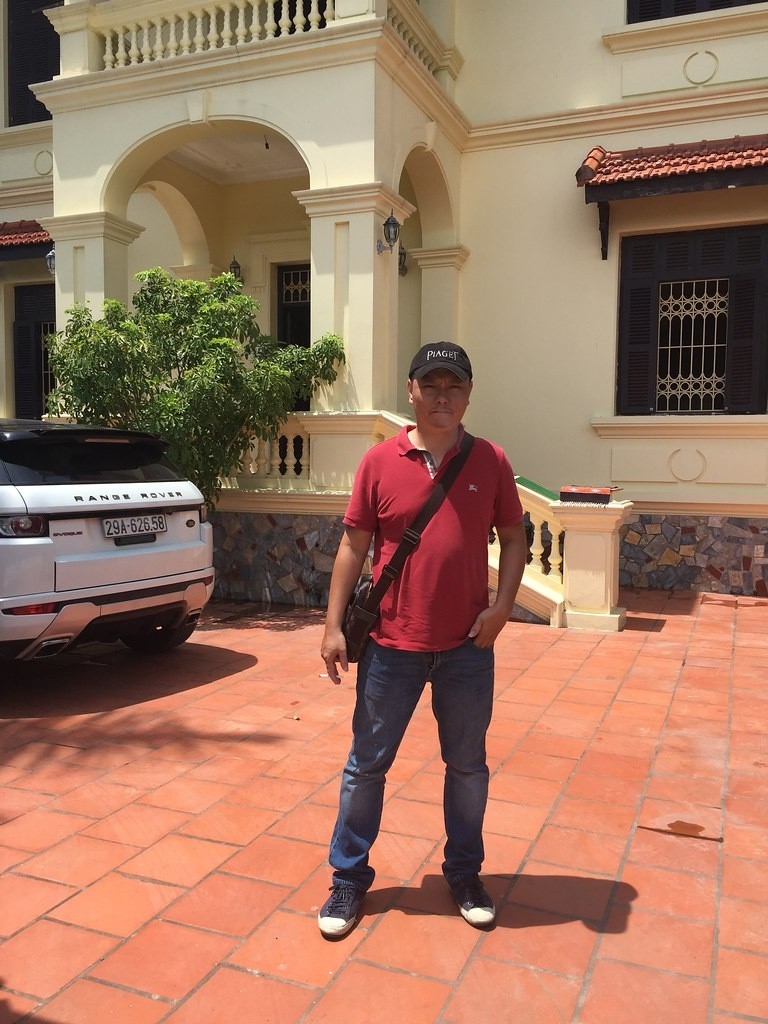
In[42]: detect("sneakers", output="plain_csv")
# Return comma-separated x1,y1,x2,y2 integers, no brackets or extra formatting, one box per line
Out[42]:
318,884,367,938
448,875,496,927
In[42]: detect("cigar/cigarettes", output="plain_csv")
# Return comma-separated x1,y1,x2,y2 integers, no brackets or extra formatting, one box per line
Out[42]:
320,674,343,680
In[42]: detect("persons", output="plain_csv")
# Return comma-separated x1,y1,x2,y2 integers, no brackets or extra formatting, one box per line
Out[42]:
319,341,527,937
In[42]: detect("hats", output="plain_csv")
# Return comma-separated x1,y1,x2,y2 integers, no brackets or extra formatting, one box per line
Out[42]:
408,341,471,381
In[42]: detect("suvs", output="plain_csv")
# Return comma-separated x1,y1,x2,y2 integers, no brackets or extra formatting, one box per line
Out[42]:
0,416,215,690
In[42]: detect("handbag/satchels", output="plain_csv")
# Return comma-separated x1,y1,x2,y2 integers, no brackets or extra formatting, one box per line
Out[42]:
337,573,379,663
